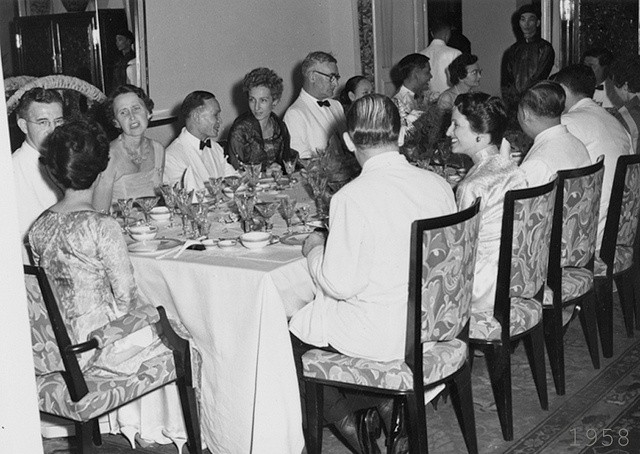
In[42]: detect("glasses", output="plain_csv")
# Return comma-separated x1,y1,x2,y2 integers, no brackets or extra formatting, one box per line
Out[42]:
25,118,64,128
467,68,484,76
314,70,341,82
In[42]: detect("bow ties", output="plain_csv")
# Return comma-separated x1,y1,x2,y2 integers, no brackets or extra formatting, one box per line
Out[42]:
318,100,330,107
595,85,604,91
199,138,210,150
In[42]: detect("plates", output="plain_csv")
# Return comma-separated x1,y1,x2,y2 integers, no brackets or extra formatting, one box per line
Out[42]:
202,239,217,246
256,178,300,188
218,239,236,247
280,233,320,245
124,238,182,256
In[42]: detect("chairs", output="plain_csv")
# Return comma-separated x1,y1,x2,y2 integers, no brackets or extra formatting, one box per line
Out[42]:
469,177,560,441
598,155,640,357
301,197,481,450
544,156,605,395
24,267,200,454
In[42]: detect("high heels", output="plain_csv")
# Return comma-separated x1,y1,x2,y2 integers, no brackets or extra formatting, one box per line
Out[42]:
120,425,157,450
161,425,206,454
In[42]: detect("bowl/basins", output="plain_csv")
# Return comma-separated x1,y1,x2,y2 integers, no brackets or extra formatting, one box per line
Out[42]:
240,232,272,250
458,168,469,177
129,225,158,240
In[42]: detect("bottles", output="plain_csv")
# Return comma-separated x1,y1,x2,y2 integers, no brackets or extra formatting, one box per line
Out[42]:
245,163,262,191
284,155,301,185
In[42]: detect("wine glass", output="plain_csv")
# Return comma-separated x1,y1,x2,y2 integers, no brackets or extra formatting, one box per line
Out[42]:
438,142,452,176
118,198,132,232
402,143,418,162
281,200,295,229
227,176,240,206
210,177,221,196
159,186,178,225
173,190,193,235
298,207,309,225
196,189,205,204
256,203,279,231
136,197,159,223
235,194,255,235
416,157,432,171
505,129,521,149
189,205,208,237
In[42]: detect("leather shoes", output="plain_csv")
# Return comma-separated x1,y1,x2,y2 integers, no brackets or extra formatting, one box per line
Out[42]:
357,408,382,454
388,397,410,454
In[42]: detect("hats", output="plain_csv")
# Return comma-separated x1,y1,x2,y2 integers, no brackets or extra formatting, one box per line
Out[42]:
519,4,539,18
117,29,134,42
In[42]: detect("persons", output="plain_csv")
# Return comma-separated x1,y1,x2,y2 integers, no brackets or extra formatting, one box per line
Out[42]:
21,117,189,454
438,54,484,112
281,51,347,160
230,68,305,175
438,10,472,55
162,91,241,203
339,75,374,117
288,93,458,453
389,53,432,146
555,63,635,252
583,48,615,108
447,92,529,314
92,85,165,215
417,16,463,103
499,4,556,132
607,53,640,155
517,79,591,188
10,88,64,265
114,28,136,87
